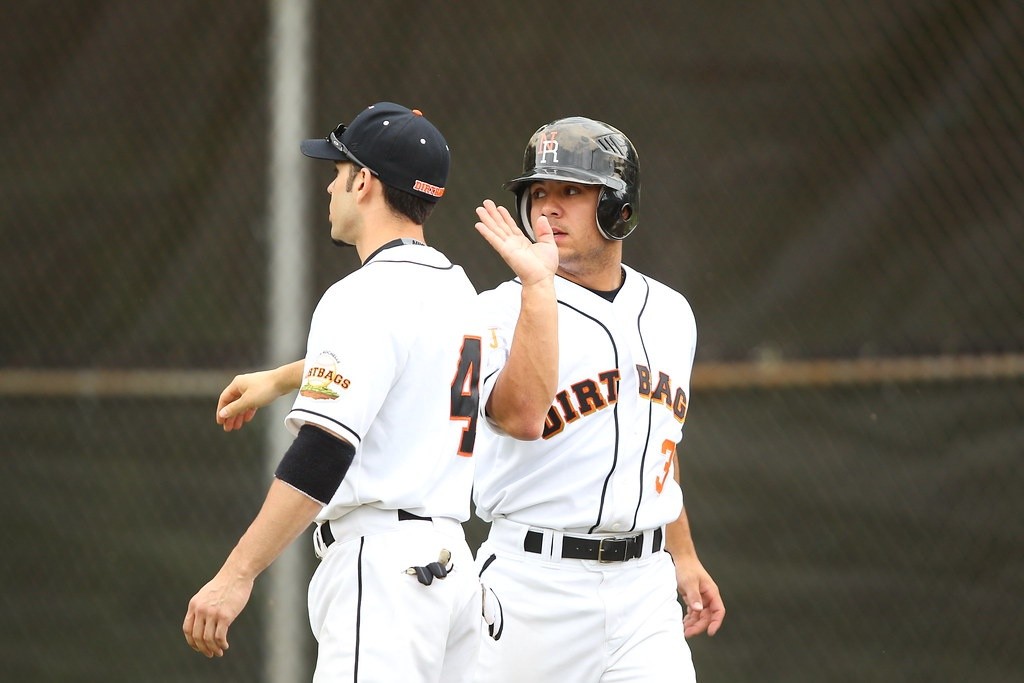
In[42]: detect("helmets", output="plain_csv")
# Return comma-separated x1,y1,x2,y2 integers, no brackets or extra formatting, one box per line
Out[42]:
501,115,641,246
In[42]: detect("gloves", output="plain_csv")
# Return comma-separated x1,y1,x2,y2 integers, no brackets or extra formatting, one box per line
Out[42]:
408,550,452,585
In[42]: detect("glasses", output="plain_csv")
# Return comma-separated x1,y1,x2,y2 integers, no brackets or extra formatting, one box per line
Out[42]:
326,122,379,177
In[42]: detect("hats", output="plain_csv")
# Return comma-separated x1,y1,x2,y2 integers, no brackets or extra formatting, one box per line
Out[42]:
300,103,450,203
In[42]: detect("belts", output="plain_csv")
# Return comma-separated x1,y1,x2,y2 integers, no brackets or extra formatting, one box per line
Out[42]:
524,528,662,562
320,507,432,546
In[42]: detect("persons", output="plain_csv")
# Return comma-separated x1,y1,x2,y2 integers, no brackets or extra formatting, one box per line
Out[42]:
182,103,484,683
475,117,725,683
